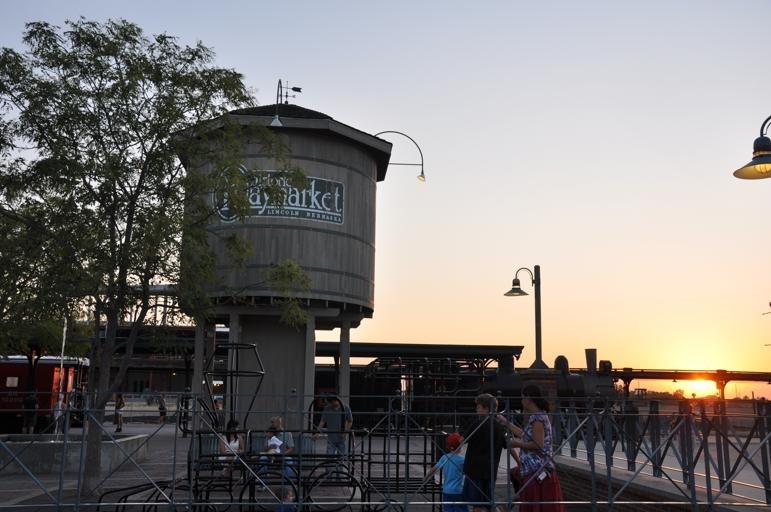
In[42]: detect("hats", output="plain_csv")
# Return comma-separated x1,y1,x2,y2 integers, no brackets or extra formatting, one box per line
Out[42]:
447,433,462,450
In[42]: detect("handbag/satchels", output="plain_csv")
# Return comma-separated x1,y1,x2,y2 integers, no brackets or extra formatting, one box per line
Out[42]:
226,449,237,460
510,466,523,493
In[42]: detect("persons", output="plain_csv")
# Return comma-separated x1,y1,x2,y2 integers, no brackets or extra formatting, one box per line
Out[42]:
219,419,244,478
159,393,166,424
144,386,159,405
115,391,125,432
212,399,220,427
54,394,66,434
592,391,640,442
275,486,297,512
315,394,353,478
21,389,39,442
256,414,296,502
495,385,566,512
423,432,469,511
459,395,521,511
263,427,283,464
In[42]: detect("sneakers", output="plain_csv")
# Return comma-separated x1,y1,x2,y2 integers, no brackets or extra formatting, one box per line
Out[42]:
257,486,266,491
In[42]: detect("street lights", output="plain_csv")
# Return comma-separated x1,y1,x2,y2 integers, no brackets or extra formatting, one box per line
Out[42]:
731,118,771,181
503,264,549,370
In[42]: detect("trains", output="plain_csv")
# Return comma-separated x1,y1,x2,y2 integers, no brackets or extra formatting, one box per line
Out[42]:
304,344,652,442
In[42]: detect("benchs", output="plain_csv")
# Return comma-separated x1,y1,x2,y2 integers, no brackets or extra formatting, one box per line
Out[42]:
186,431,322,491
